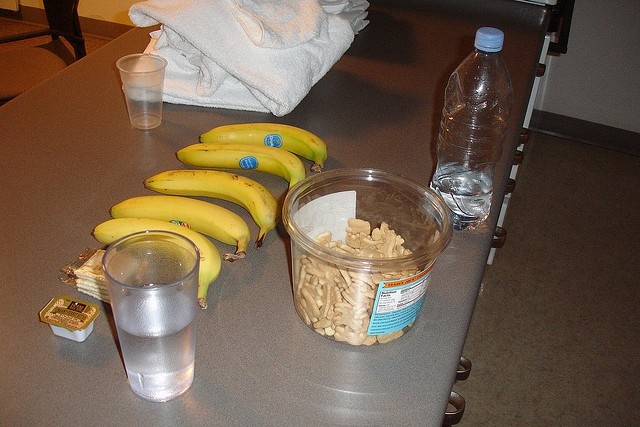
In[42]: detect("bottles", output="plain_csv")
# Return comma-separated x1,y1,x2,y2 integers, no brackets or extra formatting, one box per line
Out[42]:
429,27,513,231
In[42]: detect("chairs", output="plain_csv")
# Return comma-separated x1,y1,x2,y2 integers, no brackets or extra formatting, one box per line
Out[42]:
0,0,85,103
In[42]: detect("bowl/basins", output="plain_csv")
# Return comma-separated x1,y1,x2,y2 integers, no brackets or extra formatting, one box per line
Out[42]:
280,168,452,347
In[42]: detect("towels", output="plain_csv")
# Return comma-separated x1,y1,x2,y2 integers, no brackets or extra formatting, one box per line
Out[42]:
121,1,371,114
224,0,331,49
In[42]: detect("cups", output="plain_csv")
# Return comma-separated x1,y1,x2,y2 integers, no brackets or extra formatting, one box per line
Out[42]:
102,229,200,403
116,53,168,130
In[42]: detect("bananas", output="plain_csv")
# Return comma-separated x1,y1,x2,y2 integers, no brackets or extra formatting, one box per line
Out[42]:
143,170,282,248
175,144,307,191
90,219,222,308
199,122,328,172
109,196,251,261
